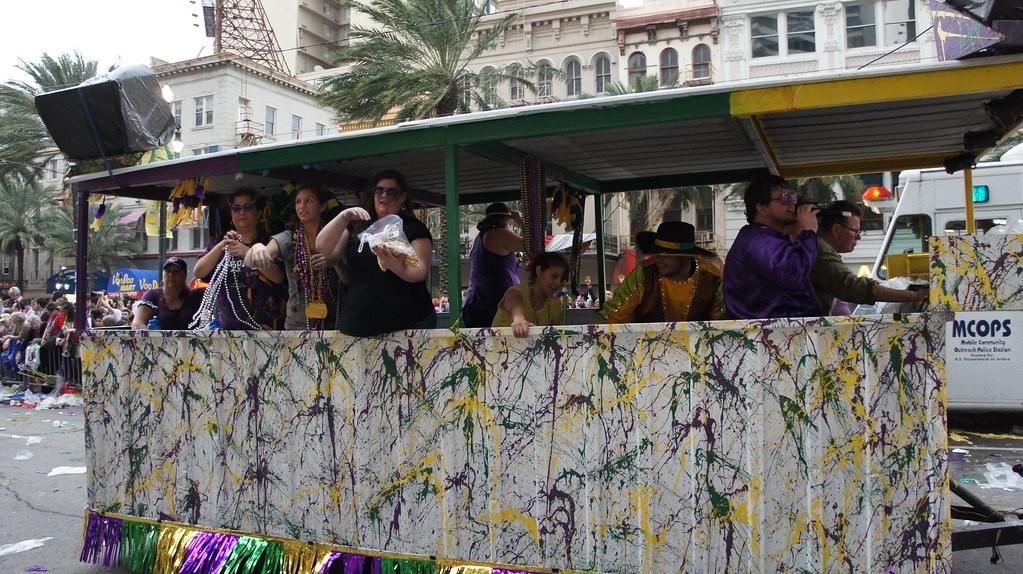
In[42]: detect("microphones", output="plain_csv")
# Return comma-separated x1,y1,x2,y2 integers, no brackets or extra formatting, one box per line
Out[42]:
810,207,852,218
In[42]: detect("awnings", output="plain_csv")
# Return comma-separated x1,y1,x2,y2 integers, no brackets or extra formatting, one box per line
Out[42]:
108,268,164,292
46,265,113,294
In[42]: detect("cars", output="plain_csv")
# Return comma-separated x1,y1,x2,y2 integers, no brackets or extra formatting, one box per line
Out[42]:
46,266,111,295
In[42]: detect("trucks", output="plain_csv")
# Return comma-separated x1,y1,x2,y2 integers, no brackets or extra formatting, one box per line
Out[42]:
851,161,1023,411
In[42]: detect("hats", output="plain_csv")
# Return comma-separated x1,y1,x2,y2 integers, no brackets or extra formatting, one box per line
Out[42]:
486,204,512,215
163,256,188,272
636,221,717,257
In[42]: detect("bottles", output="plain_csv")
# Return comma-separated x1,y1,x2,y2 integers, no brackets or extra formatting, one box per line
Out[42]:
208,315,221,330
147,316,161,330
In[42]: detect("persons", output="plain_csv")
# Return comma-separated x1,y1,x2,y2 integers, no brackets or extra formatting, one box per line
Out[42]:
315,169,437,338
723,173,820,318
0,287,157,395
814,200,930,315
586,220,725,324
462,202,526,328
491,251,570,339
244,185,343,330
132,257,201,330
557,272,626,310
193,188,286,331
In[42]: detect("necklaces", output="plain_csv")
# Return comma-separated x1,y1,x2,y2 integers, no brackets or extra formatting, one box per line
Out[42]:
188,232,263,331
529,281,551,326
293,220,341,330
419,201,428,226
519,154,547,258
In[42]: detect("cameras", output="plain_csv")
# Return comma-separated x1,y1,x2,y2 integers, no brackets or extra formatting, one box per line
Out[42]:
90,290,103,297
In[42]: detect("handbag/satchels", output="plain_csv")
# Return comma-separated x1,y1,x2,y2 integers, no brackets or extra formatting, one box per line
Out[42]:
25,343,40,368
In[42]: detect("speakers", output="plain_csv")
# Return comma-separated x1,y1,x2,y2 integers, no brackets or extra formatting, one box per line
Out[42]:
35,75,172,162
945,0,1023,34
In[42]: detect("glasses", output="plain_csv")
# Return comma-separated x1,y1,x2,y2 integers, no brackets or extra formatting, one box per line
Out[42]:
838,223,862,237
374,187,400,195
772,192,798,205
230,204,254,210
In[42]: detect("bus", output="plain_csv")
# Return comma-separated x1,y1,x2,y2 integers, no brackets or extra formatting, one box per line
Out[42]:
58,51,1023,574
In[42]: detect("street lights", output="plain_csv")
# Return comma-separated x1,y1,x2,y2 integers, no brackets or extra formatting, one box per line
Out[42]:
159,83,185,285
